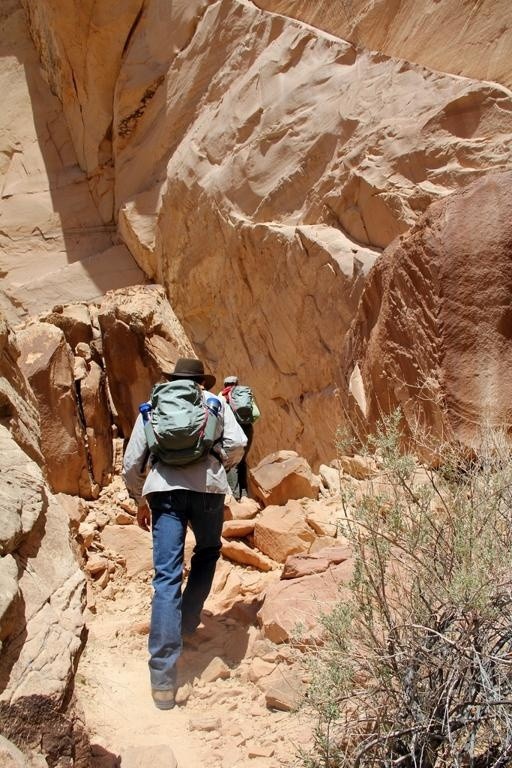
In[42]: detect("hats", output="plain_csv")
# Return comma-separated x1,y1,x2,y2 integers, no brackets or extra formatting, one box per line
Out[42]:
224,376,239,384
160,357,216,391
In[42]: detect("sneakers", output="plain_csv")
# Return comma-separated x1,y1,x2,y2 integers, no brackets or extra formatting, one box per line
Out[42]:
151,688,176,710
182,633,195,647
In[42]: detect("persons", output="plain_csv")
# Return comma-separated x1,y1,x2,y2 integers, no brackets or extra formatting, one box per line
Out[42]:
117,354,248,712
218,374,265,500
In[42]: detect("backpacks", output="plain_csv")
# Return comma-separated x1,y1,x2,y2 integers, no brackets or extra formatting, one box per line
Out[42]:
143,379,218,467
230,385,253,416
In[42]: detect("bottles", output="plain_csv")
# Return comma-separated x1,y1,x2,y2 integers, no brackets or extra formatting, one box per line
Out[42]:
207,397,221,417
139,403,154,427
